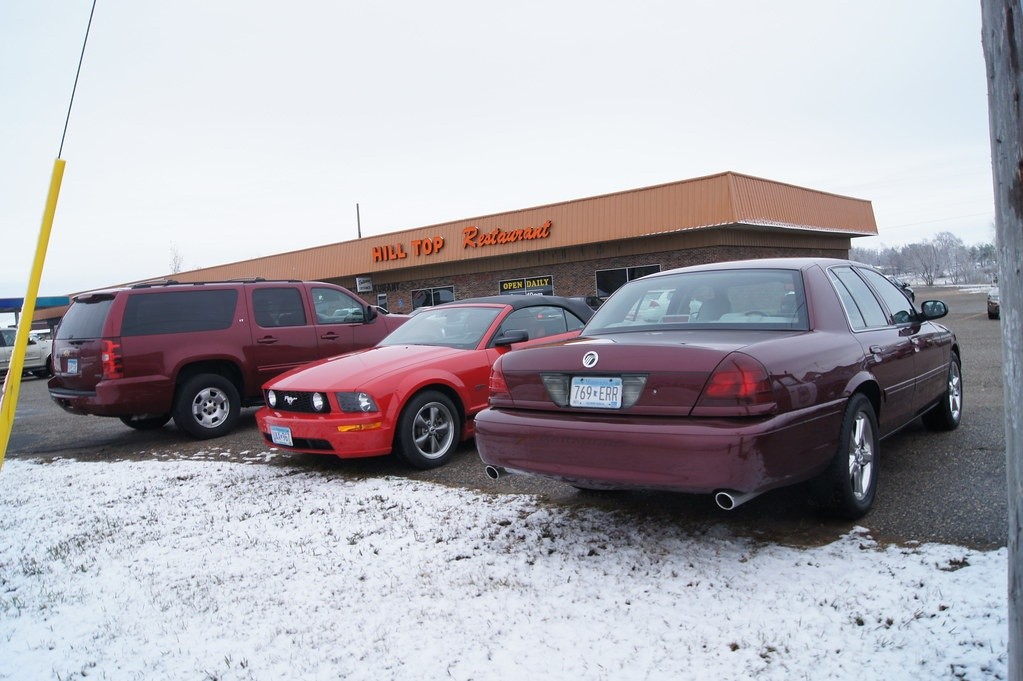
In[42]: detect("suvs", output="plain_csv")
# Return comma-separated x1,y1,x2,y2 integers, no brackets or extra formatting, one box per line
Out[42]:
47,277,415,443
0,327,56,379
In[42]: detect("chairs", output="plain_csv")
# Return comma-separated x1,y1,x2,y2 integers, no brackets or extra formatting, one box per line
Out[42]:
780,294,798,314
278,296,306,324
695,296,731,321
503,309,545,340
463,314,502,348
253,288,278,327
182,291,228,321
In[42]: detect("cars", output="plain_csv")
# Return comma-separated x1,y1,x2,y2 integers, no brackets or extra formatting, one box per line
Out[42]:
883,274,916,304
255,288,702,472
334,305,391,317
472,257,962,524
987,283,1000,320
